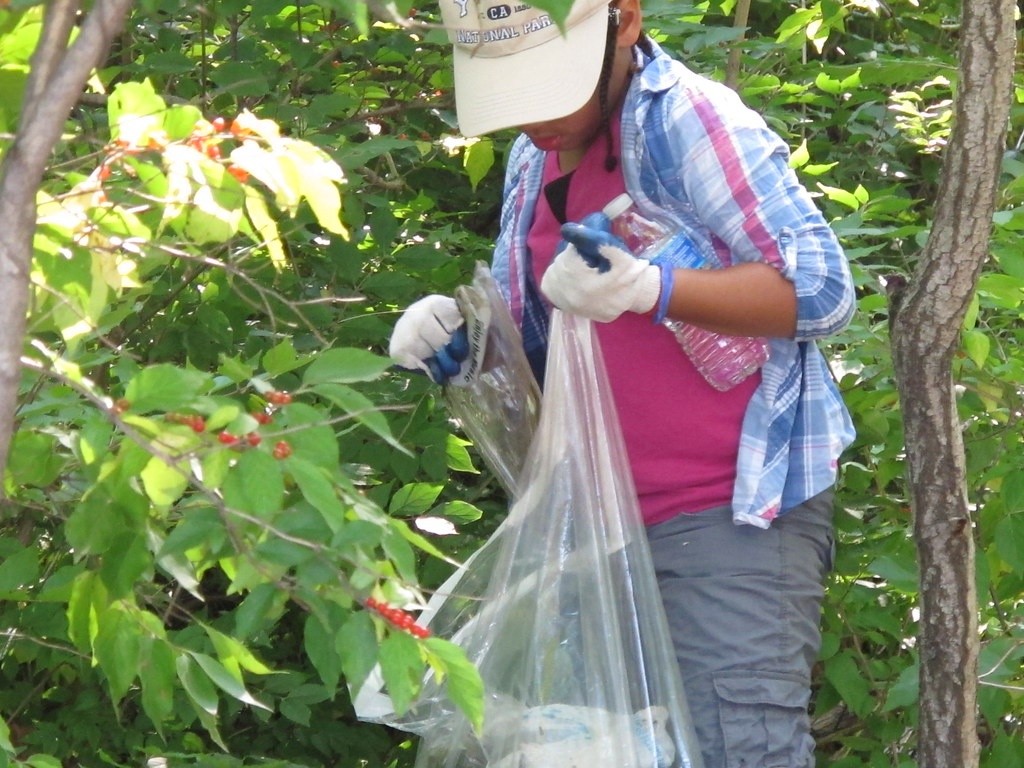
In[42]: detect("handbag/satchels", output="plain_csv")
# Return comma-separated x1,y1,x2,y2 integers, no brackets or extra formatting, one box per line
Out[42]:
351,312,700,766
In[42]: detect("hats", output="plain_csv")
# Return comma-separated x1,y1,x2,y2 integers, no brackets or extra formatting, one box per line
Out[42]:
433,0,620,138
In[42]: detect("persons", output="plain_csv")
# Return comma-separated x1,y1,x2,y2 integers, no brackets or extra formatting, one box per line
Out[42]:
387,2,855,767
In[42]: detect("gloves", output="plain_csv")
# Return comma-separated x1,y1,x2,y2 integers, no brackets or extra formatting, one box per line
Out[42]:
392,289,477,387
535,214,664,329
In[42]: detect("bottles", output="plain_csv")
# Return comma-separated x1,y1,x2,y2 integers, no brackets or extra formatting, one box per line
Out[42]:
604,191,770,394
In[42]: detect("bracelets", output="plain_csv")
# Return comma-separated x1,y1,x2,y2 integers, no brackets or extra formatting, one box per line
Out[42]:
649,256,675,321
